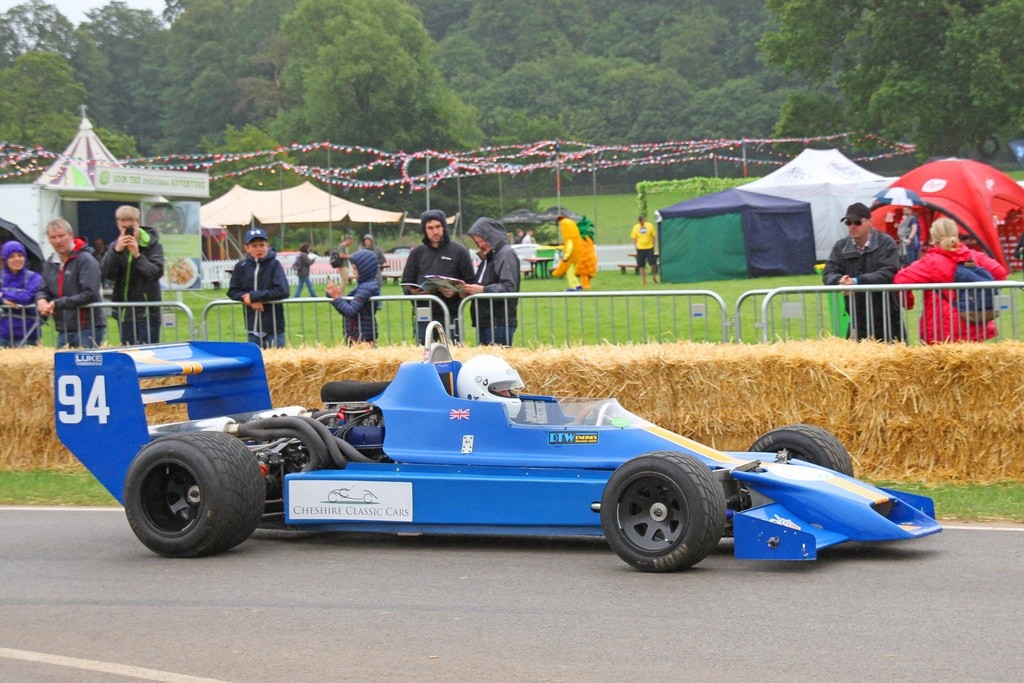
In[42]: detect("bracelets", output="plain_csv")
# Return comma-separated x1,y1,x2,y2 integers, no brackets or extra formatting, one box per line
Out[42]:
907,238,912,241
853,277,858,285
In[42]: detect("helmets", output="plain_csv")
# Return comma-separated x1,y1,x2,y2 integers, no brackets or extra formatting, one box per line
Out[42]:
456,353,525,418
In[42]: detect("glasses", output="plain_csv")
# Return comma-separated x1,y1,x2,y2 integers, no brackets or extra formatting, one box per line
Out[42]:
845,220,868,226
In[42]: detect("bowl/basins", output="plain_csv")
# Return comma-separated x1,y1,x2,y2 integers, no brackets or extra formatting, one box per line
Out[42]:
143,204,182,236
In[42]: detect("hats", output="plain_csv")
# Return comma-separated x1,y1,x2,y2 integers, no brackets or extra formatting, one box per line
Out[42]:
244,228,268,244
842,204,872,222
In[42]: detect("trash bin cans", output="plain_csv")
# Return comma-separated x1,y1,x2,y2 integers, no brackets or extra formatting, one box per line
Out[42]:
813,264,850,338
536,248,562,279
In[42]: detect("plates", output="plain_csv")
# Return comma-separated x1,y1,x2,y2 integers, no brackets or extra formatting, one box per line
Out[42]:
159,258,197,290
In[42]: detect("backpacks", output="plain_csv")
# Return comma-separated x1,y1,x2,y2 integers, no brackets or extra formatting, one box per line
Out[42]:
330,248,343,267
291,255,303,270
938,253,1001,323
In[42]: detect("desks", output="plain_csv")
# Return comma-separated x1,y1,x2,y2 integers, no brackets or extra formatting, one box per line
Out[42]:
524,257,554,279
629,253,659,274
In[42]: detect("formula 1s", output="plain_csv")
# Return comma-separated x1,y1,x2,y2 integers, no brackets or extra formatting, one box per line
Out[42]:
53,339,946,573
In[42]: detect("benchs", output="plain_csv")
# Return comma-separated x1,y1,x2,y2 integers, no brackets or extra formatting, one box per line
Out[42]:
618,266,640,275
380,273,401,286
348,276,360,285
521,269,535,280
548,268,557,277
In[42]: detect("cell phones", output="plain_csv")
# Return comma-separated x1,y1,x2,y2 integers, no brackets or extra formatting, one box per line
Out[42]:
125,227,134,236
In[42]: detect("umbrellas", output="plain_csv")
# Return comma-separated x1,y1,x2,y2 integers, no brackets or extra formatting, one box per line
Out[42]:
872,187,926,225
498,208,543,225
525,205,583,224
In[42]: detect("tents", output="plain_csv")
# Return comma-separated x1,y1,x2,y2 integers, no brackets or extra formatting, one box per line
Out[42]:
655,147,1023,275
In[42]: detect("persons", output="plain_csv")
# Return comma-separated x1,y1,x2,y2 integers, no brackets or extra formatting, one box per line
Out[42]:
0,202,656,345
895,216,1006,347
457,353,597,427
822,202,907,343
